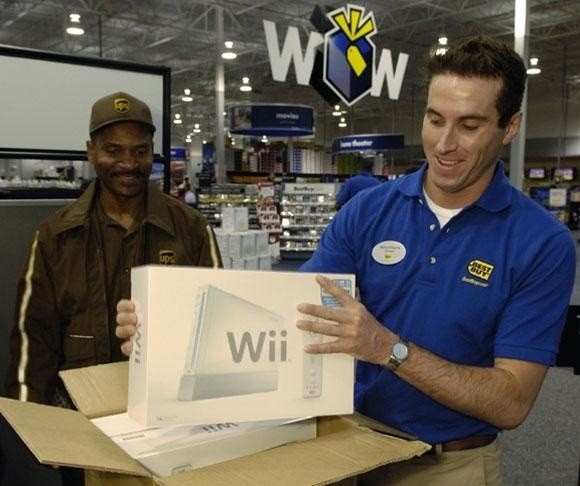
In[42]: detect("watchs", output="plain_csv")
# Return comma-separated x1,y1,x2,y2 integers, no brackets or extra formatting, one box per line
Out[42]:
385,331,410,377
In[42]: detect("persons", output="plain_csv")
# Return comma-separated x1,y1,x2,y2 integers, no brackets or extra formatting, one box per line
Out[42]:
5,90,223,485
115,36,575,486
178,176,192,190
193,171,201,197
334,156,384,212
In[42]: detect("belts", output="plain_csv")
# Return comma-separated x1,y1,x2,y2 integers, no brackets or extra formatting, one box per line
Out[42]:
428,435,495,455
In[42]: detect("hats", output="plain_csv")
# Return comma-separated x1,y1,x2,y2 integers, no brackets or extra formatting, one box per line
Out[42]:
89,92,156,133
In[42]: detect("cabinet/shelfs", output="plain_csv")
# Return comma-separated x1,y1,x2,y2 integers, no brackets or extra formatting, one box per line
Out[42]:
197,179,342,261
